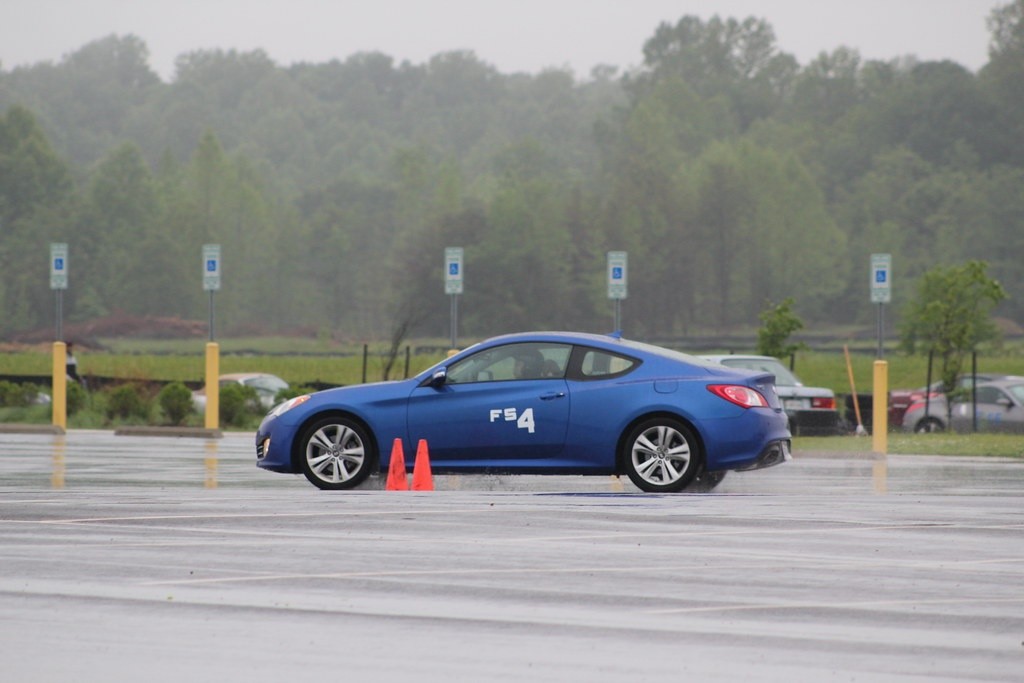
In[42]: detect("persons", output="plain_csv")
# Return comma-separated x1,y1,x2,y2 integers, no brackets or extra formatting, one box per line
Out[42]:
63,341,81,384
513,350,545,378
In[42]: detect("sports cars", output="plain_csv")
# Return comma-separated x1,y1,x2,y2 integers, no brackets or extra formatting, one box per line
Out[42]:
255,330,793,495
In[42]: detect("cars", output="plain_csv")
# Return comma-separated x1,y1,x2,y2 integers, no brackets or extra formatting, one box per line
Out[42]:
839,373,1024,435
189,371,289,413
694,353,838,435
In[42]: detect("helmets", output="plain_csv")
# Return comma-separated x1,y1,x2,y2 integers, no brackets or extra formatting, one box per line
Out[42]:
513,349,544,378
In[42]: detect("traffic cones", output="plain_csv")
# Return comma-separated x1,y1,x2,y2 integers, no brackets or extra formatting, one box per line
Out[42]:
407,431,445,492
384,438,410,491
